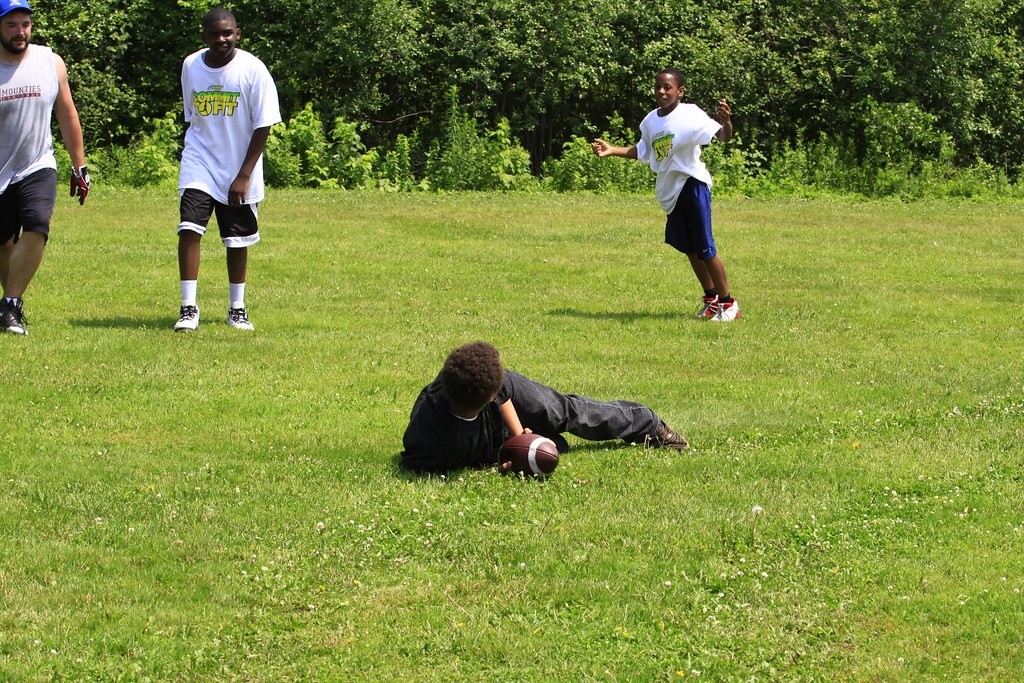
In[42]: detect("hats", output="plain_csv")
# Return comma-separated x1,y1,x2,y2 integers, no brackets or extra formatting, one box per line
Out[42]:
0,0,34,17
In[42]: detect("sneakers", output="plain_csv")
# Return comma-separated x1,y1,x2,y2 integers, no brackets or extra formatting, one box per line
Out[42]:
227,306,254,331
648,420,689,449
693,295,718,320
710,298,741,322
0,296,30,336
174,305,200,331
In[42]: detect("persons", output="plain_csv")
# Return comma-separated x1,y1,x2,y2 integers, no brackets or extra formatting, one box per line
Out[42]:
591,67,742,322
0,0,90,335
173,8,283,331
400,342,690,476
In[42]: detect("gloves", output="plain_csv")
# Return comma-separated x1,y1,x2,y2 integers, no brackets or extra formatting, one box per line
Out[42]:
69,164,90,206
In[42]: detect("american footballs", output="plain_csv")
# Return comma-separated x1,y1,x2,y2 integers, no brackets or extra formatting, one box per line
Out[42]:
498,435,559,479
